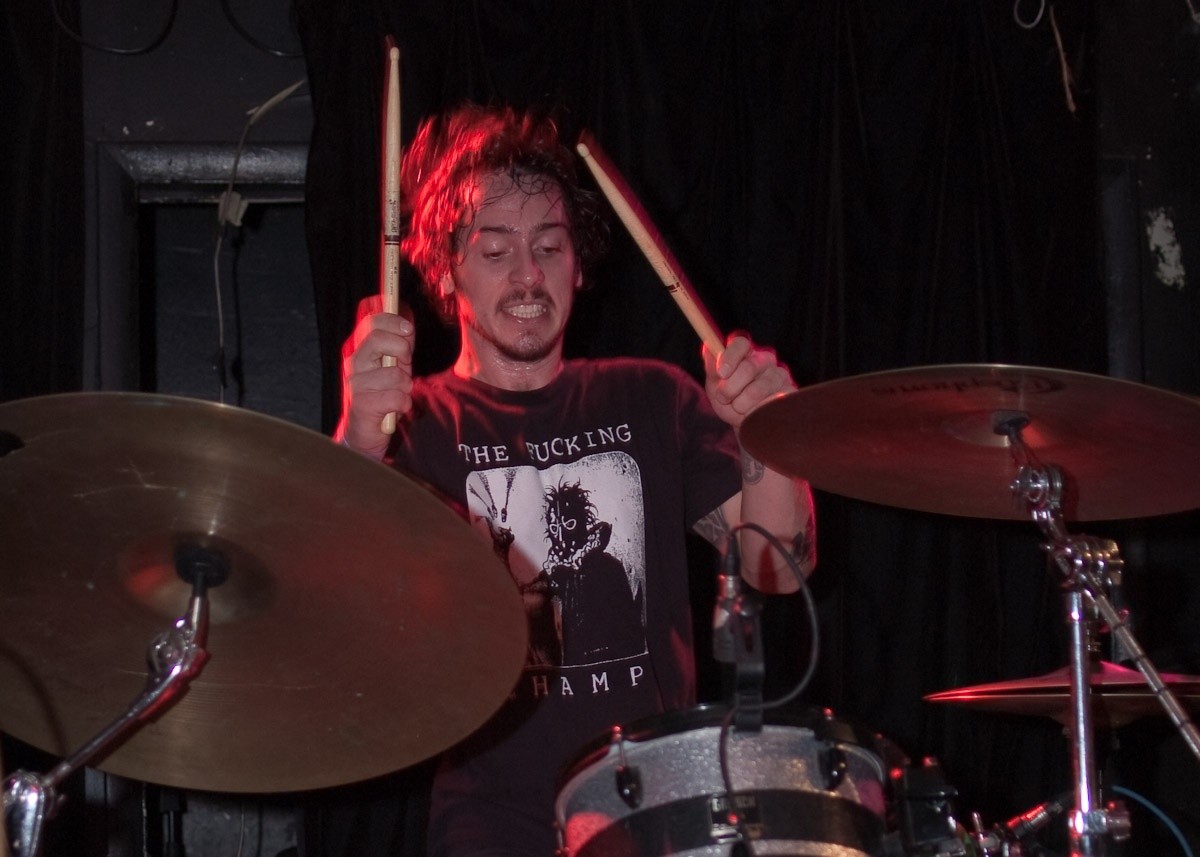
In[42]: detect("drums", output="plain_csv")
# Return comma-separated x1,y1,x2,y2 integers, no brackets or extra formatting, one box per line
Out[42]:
549,697,896,857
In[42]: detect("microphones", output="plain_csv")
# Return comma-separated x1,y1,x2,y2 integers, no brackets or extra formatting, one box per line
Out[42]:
979,791,1075,853
711,534,742,663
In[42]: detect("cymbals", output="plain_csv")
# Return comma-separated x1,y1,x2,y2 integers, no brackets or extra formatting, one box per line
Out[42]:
921,655,1200,736
736,361,1200,524
1,388,532,795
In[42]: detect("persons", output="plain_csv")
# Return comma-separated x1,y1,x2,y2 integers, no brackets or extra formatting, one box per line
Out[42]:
332,108,817,857
